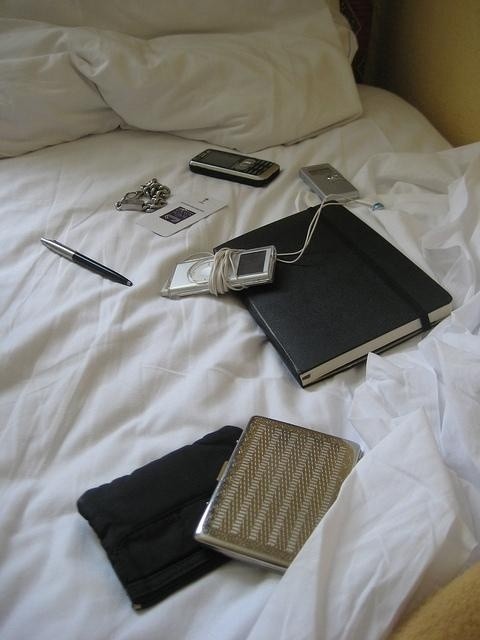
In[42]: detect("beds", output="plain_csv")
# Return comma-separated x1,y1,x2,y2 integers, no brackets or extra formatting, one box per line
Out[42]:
1,0,480,639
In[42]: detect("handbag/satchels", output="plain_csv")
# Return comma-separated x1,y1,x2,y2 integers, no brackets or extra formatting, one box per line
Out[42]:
77,421,248,611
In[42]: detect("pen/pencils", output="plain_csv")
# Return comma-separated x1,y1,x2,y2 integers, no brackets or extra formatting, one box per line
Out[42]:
39,238,133,286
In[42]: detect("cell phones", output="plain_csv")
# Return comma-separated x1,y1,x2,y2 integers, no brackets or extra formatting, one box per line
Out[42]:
189,149,280,187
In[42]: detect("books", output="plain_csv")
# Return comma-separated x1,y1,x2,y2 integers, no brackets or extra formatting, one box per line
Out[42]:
214,201,457,389
